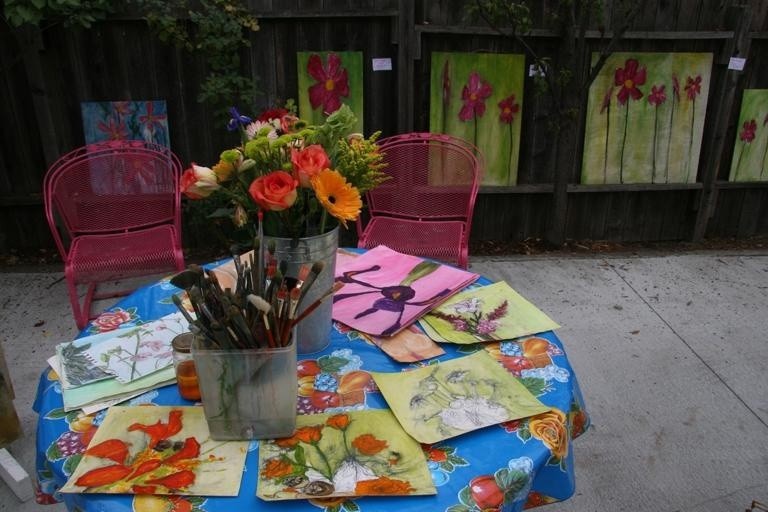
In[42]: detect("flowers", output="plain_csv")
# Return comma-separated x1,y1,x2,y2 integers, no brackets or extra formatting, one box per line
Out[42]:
179,101,398,238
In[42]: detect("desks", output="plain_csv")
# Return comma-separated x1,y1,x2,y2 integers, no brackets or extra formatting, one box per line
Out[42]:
36,246,573,512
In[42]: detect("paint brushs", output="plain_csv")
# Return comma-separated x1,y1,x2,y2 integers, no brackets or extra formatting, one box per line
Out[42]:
170,237,346,348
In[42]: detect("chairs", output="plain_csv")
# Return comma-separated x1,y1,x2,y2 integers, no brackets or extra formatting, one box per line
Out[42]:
353,133,487,270
40,137,186,330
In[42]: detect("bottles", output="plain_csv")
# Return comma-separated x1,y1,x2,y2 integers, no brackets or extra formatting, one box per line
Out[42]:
171,333,201,402
